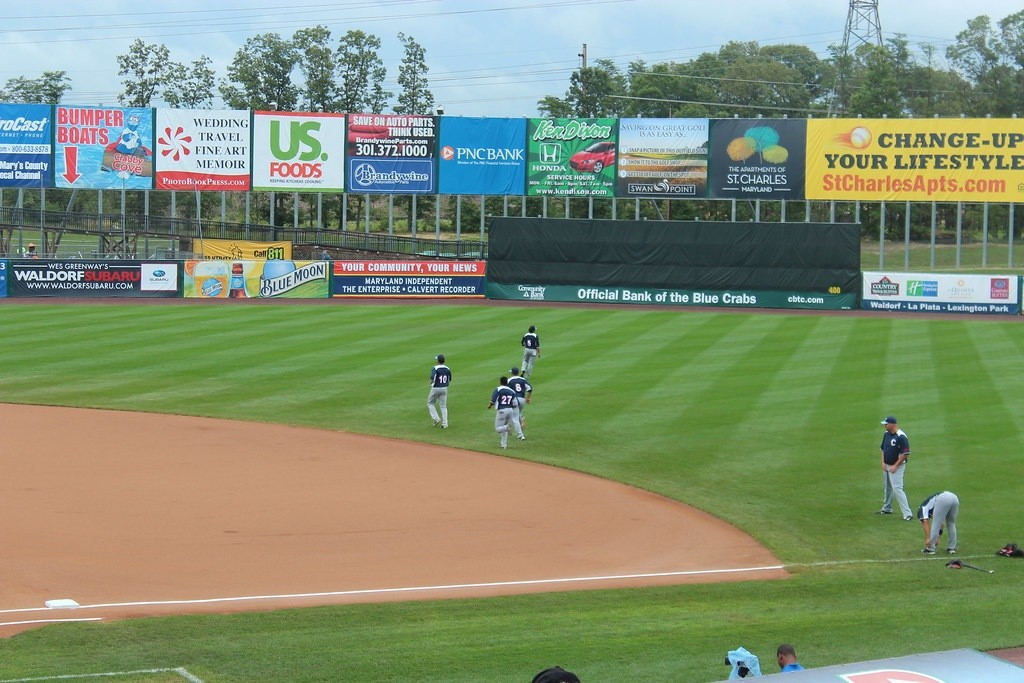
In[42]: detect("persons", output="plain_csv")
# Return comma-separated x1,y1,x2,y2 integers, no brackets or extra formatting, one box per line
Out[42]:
507,367,532,429
777,644,805,673
488,377,520,449
521,326,540,379
25,242,37,258
880,416,914,521
427,354,452,428
916,491,960,555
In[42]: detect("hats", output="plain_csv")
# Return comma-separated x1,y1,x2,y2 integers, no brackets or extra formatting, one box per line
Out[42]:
532,667,582,683
434,355,446,363
528,326,537,333
881,415,897,425
508,367,520,375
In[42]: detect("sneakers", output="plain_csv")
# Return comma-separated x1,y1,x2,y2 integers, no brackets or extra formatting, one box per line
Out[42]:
499,445,507,449
905,516,913,521
875,511,890,514
517,437,525,441
442,425,448,429
521,419,526,429
947,549,956,554
431,418,441,427
921,549,936,555
520,370,526,377
507,422,512,436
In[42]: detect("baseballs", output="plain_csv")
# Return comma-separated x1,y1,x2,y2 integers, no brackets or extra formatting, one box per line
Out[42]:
850,127,871,148
